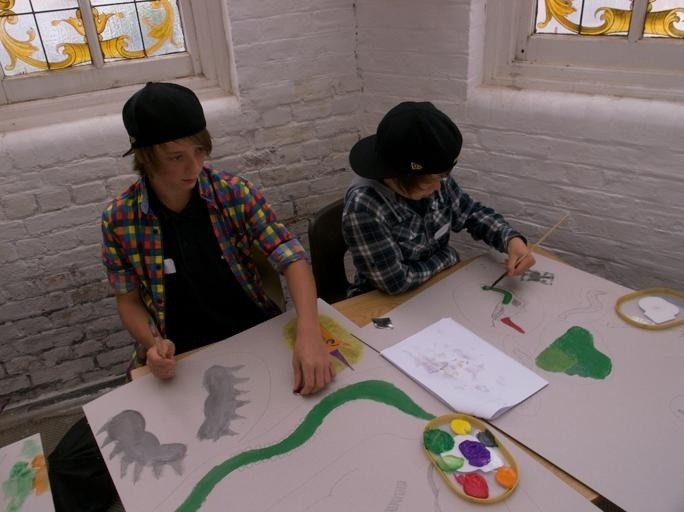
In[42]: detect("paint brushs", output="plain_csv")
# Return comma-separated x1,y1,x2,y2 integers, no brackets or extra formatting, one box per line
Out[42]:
490,213,573,291
148,318,166,360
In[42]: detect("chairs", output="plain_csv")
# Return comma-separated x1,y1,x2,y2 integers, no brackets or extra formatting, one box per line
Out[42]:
308,197,358,304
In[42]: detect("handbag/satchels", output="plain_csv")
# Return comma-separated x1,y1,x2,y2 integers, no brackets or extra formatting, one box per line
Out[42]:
48,416,118,512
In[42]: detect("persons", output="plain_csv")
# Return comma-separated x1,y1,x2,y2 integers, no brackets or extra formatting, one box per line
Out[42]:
336,98,537,295
97,81,336,400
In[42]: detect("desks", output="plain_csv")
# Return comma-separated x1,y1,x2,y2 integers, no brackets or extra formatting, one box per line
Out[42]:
129,243,607,505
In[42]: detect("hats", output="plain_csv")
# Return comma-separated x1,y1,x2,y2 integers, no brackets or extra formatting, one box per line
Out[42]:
122,81,207,158
349,100,463,179
